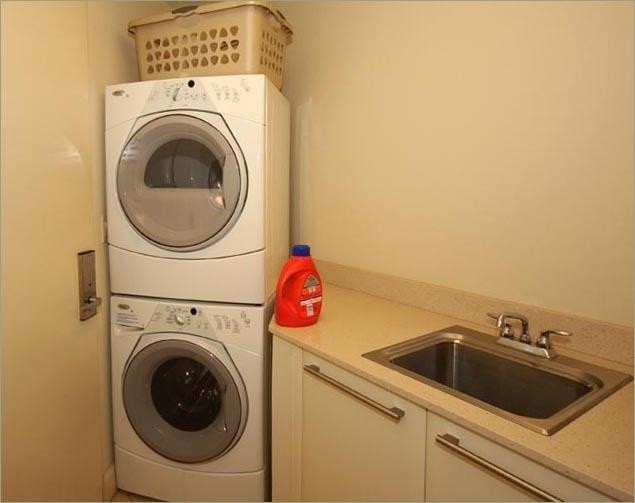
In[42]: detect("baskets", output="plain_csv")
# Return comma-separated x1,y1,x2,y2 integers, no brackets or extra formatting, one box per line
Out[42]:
125,0,294,92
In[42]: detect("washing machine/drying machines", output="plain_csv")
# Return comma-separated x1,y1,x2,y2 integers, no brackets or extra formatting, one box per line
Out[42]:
110,296,275,502
102,74,289,305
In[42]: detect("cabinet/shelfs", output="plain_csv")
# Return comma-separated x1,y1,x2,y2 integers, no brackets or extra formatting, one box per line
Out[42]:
267,327,620,503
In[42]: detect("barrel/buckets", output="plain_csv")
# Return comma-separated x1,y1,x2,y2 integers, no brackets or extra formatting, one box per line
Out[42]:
274,244,323,328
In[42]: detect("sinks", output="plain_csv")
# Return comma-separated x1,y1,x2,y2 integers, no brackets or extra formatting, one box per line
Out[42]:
359,325,634,437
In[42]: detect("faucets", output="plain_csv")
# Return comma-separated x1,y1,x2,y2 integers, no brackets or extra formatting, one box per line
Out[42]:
495,311,530,342
